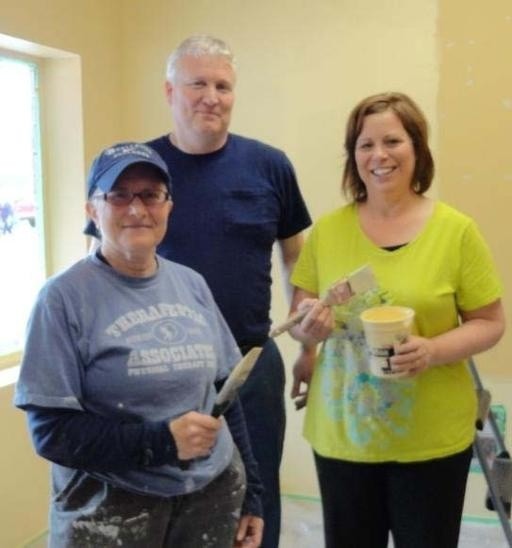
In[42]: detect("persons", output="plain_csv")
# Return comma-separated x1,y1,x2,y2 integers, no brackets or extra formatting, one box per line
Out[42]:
80,31,320,548
12,139,269,548
287,84,508,548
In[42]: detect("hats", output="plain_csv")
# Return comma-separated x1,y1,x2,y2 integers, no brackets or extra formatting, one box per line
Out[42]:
87,142,171,199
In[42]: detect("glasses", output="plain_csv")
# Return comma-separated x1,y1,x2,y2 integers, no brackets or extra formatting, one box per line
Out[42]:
93,189,172,205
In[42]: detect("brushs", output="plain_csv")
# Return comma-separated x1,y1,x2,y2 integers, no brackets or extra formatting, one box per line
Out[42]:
267,263,377,339
179,347,264,471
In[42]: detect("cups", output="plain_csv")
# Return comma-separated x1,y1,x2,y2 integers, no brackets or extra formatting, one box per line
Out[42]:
359,303,416,377
